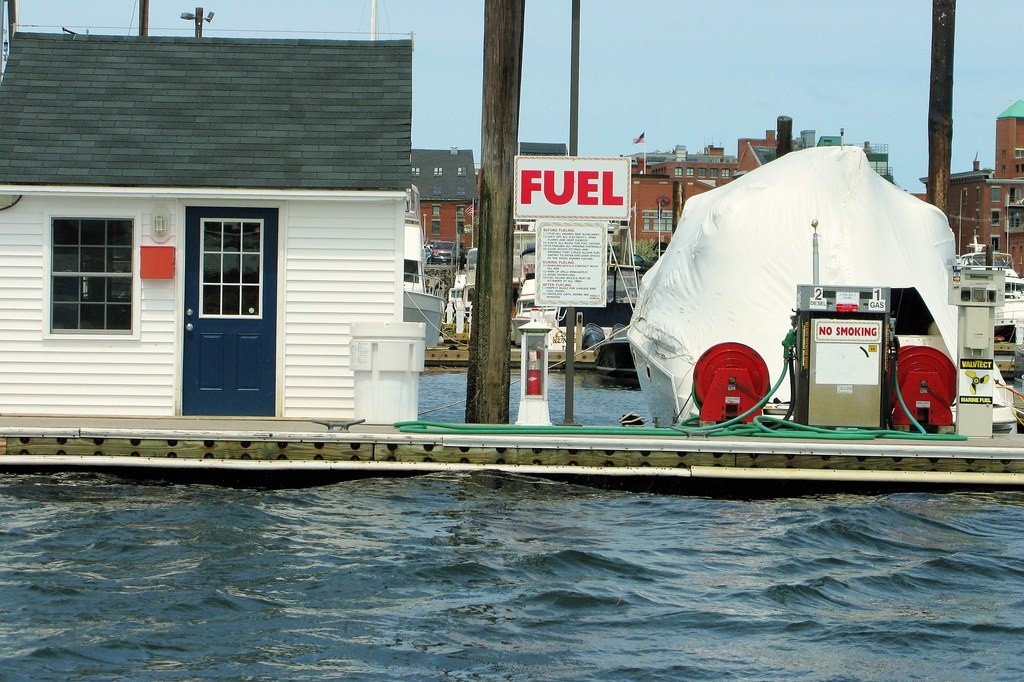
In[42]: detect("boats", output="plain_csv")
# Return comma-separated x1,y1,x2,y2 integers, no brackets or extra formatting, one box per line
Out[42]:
560,200,662,372
404,184,445,348
510,247,561,348
955,189,1024,380
444,194,479,339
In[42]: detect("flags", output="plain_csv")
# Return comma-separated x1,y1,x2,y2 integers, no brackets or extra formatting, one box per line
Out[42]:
633,132,644,143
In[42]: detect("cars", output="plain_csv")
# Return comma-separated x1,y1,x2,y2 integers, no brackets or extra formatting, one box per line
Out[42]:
432,241,455,264
